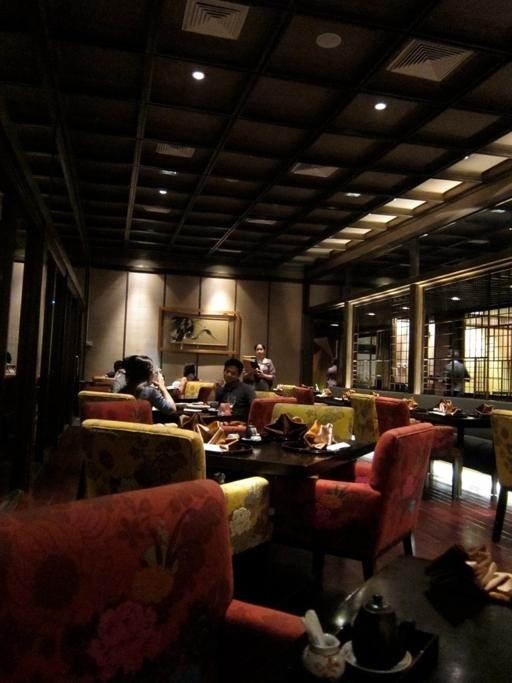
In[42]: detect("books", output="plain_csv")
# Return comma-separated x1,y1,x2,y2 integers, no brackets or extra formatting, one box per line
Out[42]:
243,355,261,369
242,358,260,374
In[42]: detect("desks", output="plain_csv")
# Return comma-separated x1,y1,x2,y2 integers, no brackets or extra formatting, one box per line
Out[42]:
202,440,384,583
281,555,512,683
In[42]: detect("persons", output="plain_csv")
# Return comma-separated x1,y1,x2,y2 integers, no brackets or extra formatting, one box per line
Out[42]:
118,354,181,428
104,360,121,375
244,341,276,391
113,364,128,393
437,348,471,396
206,358,256,420
177,360,198,399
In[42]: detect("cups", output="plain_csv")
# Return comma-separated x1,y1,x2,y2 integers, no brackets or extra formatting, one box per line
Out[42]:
226,395,236,409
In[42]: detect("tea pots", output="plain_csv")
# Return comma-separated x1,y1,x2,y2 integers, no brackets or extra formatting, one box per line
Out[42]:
345,591,418,671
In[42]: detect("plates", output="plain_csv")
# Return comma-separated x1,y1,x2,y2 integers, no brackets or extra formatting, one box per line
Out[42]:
183,406,218,416
340,640,414,674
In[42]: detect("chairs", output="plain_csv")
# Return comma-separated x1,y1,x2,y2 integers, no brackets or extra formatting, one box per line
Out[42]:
78,374,501,503
308,420,435,582
1,477,308,682
80,415,275,584
489,407,512,546
